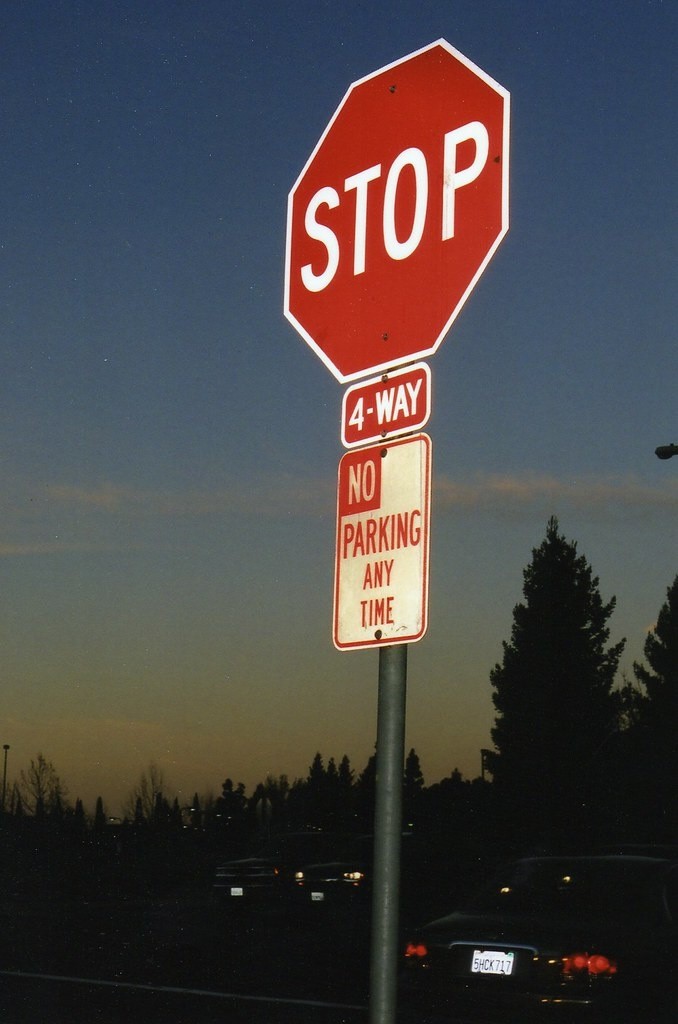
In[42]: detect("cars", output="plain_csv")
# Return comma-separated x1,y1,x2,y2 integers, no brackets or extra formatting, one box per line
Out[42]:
407,855,678,1024
215,831,362,911
283,832,498,923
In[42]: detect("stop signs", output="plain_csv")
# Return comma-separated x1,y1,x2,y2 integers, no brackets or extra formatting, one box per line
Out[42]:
282,38,512,384
